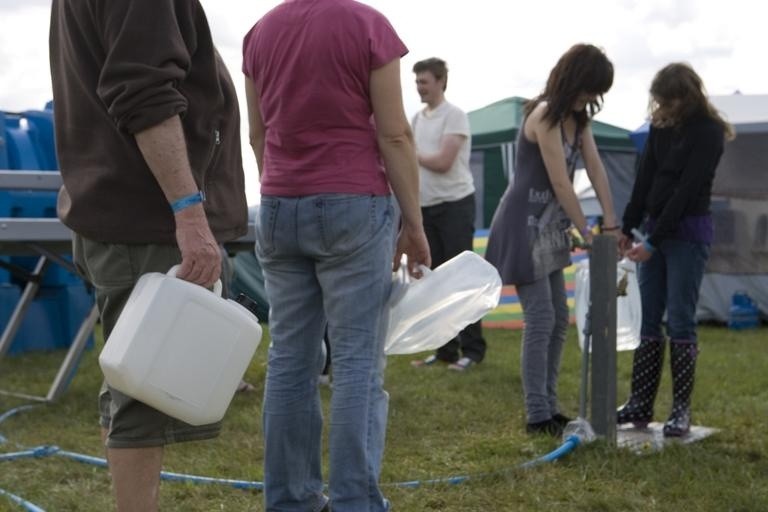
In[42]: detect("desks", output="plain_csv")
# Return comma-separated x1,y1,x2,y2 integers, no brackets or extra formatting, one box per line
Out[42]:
0,219,256,406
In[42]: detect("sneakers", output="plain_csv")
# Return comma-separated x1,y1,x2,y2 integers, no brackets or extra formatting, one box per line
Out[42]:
449,357,481,372
411,355,444,369
237,381,260,393
554,415,576,426
526,418,564,435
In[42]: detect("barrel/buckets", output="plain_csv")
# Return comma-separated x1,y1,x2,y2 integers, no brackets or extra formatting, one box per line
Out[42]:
96,262,264,429
572,257,645,354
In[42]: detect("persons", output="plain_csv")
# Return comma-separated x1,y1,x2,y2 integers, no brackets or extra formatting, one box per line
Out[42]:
242,0,432,512
484,43,627,436
411,58,487,370
616,63,736,438
48,0,247,512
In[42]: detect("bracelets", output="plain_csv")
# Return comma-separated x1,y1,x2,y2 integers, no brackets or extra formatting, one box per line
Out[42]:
641,237,656,254
170,190,208,211
601,226,619,233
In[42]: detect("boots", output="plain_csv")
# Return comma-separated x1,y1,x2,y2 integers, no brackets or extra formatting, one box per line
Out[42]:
616,335,664,428
666,338,699,440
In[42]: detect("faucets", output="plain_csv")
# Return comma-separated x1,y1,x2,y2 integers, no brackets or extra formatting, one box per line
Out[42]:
614,266,634,298
